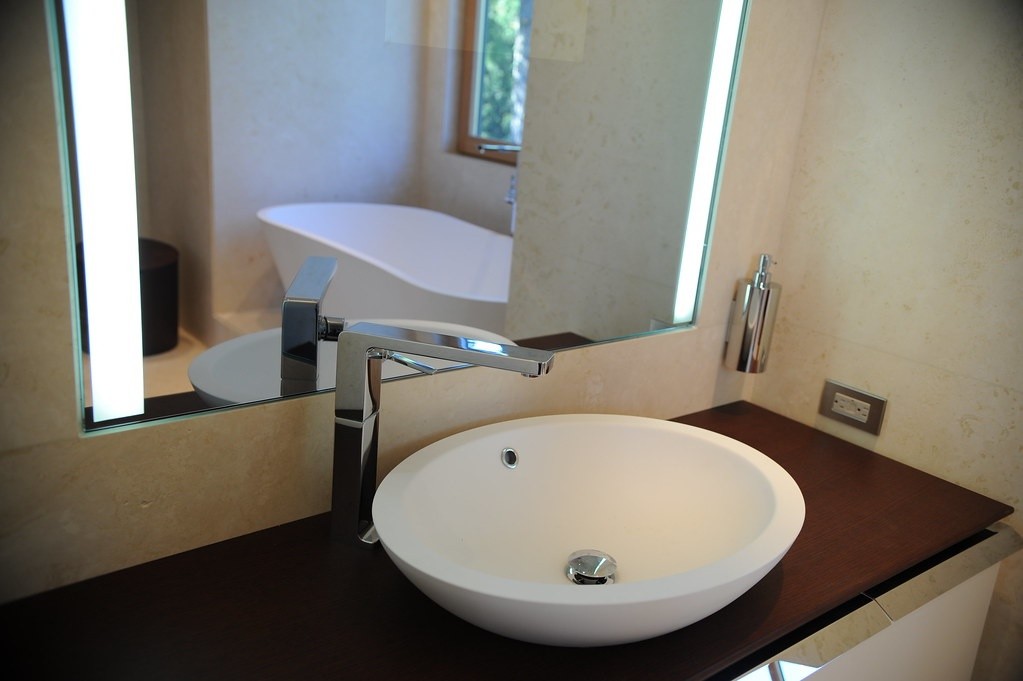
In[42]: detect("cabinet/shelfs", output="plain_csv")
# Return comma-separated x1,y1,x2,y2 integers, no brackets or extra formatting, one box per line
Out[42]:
738,525,1023,680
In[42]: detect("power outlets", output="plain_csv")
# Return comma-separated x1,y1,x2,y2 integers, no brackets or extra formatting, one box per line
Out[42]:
818,377,888,437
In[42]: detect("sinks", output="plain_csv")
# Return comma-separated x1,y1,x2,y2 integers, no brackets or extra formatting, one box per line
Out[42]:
371,413,805,647
188,320,519,408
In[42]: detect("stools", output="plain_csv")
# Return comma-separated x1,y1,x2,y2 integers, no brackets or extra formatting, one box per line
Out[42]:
77,236,179,357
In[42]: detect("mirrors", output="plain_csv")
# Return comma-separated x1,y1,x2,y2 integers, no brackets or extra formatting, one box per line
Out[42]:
44,0,755,438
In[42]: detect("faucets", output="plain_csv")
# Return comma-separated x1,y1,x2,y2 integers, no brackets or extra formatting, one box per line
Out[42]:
281,256,348,397
331,322,555,544
479,144,521,236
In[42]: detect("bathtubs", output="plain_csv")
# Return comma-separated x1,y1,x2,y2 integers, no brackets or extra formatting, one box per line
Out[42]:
255,202,514,337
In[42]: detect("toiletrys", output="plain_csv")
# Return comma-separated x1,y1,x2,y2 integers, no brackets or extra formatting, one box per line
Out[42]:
722,254,782,373
